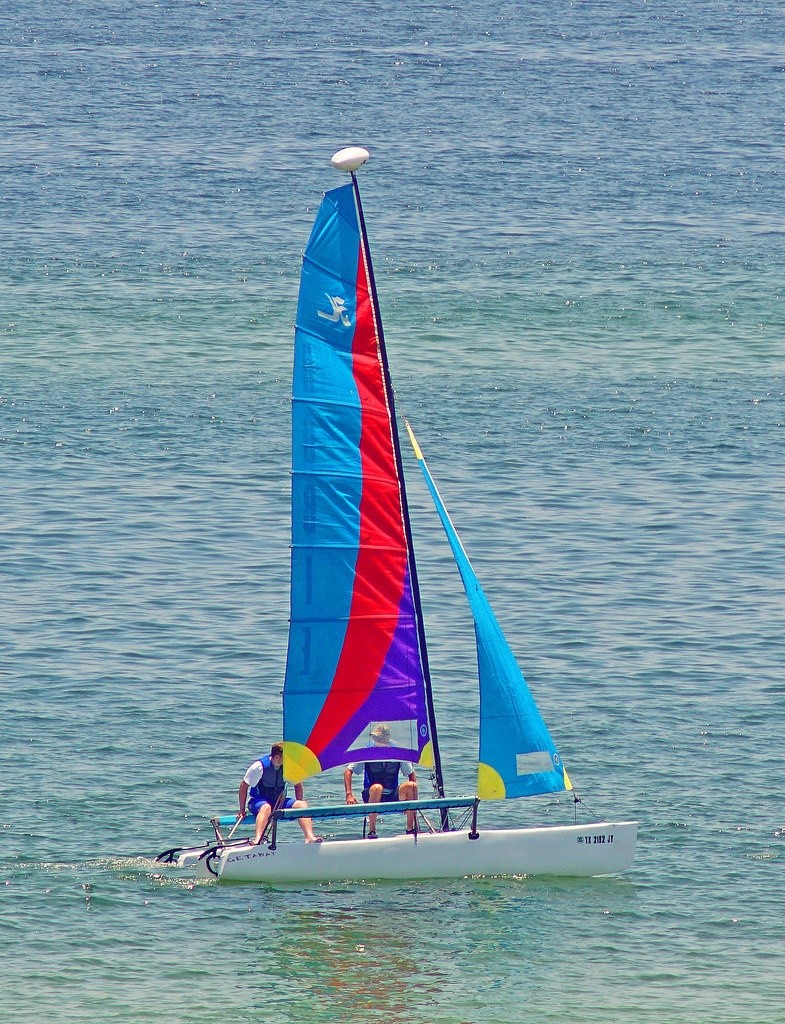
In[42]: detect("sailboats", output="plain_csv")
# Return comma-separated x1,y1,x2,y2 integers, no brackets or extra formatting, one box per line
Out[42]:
154,147,639,882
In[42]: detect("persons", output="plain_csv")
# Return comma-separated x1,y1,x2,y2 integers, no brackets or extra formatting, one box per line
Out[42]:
236,742,324,844
344,724,418,839
